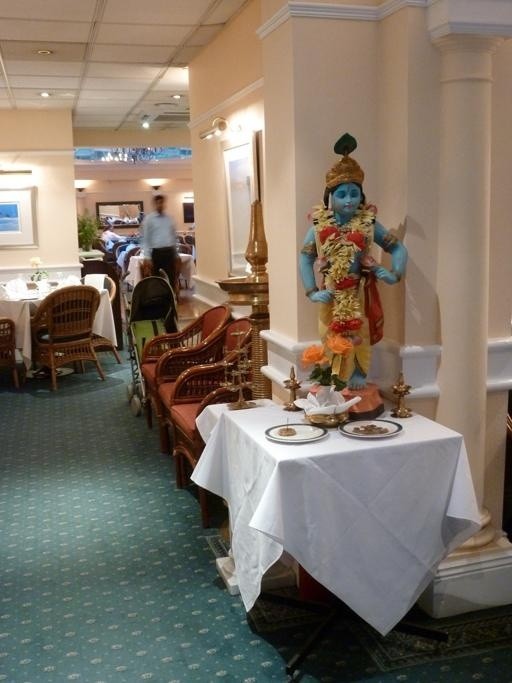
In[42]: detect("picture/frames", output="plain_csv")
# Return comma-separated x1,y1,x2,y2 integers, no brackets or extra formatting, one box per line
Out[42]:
1,187,38,250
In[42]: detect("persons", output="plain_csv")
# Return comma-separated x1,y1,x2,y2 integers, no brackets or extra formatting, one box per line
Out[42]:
142,195,184,305
299,132,409,391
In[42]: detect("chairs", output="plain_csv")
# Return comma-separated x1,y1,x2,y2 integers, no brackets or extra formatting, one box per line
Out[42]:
96,234,195,289
170,341,254,530
80,274,122,372
140,305,233,453
0,318,23,392
29,284,105,391
156,317,254,489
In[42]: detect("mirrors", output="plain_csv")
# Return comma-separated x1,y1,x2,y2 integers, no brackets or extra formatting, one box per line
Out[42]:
222,144,252,277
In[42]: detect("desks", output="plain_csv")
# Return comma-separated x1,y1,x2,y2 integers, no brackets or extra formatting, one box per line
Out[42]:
194,405,484,676
194,399,280,445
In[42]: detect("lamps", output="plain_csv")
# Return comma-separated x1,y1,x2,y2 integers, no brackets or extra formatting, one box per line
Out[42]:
77,187,87,193
199,117,229,141
152,185,161,192
101,147,163,164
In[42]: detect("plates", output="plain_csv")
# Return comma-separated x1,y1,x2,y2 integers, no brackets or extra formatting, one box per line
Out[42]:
337,419,403,440
263,423,329,443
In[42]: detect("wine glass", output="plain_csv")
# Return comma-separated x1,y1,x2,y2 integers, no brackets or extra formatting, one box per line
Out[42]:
55,271,64,284
15,273,26,281
